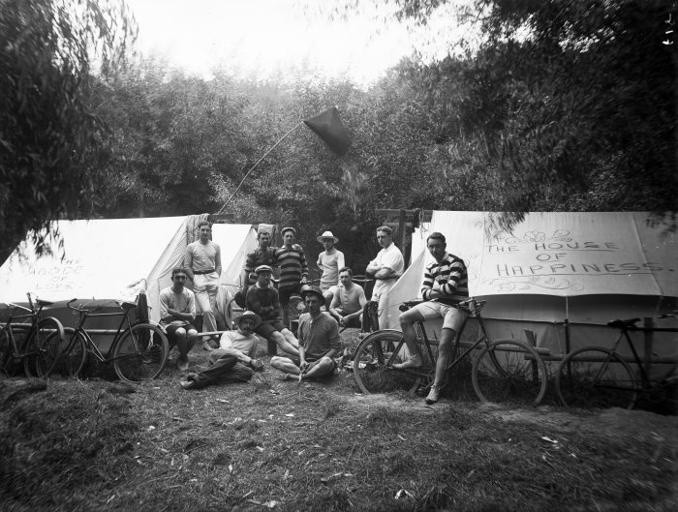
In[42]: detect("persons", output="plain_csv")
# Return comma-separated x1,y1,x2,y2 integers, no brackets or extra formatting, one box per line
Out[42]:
319,265,365,337
388,229,471,408
242,264,301,358
363,225,408,370
269,284,343,387
270,224,309,341
177,309,265,392
238,228,302,293
314,229,345,296
181,219,223,352
158,265,199,374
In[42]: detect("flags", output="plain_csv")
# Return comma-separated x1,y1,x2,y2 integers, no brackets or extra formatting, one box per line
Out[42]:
299,102,353,159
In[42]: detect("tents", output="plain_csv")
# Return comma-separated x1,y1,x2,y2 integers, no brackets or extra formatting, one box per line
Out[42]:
0,204,234,352
372,205,678,390
210,221,276,296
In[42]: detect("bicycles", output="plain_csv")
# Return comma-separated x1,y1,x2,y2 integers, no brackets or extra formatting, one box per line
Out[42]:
222,267,306,336
36,295,173,388
342,293,551,411
553,308,678,414
0,297,67,381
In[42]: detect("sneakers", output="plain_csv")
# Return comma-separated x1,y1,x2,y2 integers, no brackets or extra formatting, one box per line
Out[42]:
391,353,422,369
423,384,442,404
178,372,200,389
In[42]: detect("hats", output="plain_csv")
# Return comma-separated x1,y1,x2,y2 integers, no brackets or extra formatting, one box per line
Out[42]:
254,265,273,273
300,288,326,306
316,230,339,245
233,311,262,330
